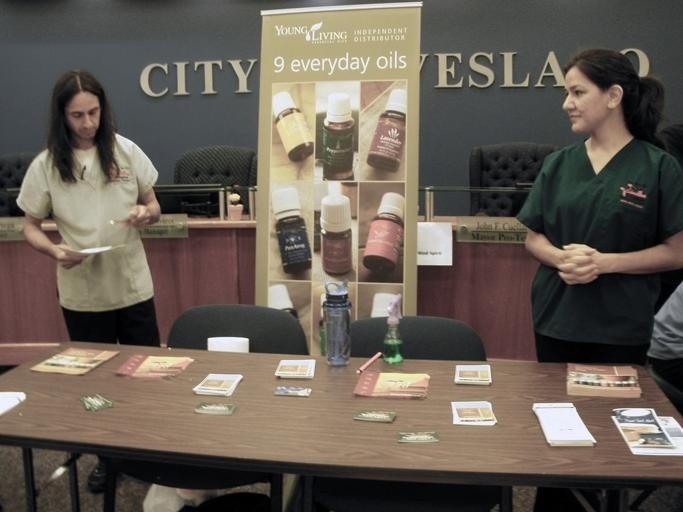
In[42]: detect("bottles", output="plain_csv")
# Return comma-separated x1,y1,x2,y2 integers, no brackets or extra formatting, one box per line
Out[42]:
320,194,355,278
320,282,354,367
321,92,357,182
367,87,405,173
272,92,314,162
270,185,312,275
268,283,299,318
361,191,404,276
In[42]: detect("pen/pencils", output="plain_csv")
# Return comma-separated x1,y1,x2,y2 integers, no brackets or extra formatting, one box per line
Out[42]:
356,351,383,374
108,219,133,225
81,394,113,410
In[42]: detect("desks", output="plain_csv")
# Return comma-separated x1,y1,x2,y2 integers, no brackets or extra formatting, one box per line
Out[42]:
0,339,682,512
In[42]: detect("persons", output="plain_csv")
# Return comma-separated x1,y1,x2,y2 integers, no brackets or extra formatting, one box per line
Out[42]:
645,282,683,394
16,70,161,494
515,49,683,512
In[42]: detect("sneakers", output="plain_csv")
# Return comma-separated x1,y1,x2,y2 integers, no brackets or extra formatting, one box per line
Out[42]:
87,459,115,492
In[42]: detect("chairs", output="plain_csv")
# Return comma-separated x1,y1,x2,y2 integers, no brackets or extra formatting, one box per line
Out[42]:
0,153,34,217
467,141,562,219
287,313,514,512
171,146,259,210
98,298,307,511
